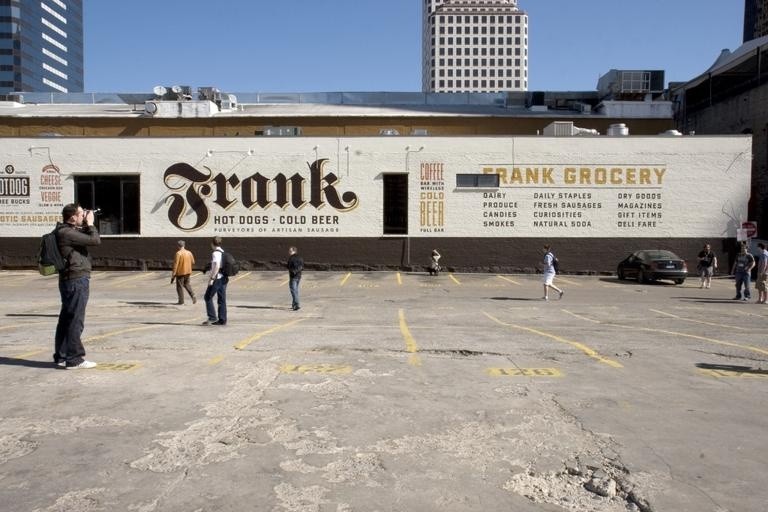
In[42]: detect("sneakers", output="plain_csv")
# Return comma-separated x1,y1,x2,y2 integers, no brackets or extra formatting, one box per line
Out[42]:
292,302,300,310
701,284,710,288
429,273,438,276
176,296,197,305
734,296,768,304
542,291,563,301
203,318,226,325
56,359,96,369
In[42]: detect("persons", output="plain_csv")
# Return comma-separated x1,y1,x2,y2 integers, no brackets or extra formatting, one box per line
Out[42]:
172,239,197,304
54,204,102,369
755,243,768,304
202,236,229,327
426,250,441,275
540,245,563,299
286,247,304,310
731,243,756,300
696,243,718,288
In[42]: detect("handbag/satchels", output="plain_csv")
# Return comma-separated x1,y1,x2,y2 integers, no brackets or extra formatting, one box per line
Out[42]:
289,264,303,275
696,264,706,274
434,265,441,271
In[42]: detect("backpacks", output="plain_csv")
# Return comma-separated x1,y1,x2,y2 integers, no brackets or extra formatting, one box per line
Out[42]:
36,226,77,276
548,254,560,272
211,250,240,276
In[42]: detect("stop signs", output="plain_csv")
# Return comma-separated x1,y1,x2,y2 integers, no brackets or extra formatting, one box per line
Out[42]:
740,222,756,238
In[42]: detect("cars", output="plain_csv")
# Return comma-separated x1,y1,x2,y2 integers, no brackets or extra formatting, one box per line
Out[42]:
616,250,688,284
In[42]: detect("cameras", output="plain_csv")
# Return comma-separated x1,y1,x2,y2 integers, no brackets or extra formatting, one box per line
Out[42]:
84,207,101,221
